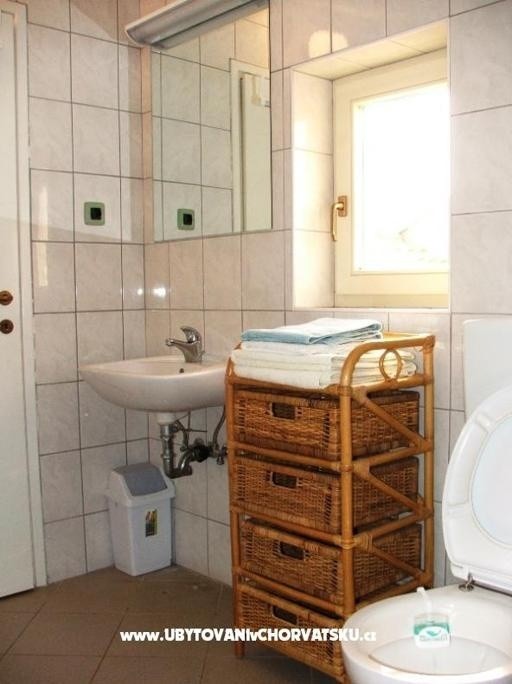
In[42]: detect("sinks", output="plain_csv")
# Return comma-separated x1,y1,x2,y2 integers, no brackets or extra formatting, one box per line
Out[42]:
79,355,229,413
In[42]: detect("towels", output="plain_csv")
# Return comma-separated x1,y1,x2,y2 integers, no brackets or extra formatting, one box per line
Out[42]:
229,316,418,393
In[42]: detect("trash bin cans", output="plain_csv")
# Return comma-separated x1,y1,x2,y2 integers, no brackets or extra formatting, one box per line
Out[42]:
102,459,177,577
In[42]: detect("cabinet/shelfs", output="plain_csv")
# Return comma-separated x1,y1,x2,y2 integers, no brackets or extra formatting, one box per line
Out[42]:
222,312,435,683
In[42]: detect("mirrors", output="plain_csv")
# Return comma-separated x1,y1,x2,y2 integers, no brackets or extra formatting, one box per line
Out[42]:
143,0,278,243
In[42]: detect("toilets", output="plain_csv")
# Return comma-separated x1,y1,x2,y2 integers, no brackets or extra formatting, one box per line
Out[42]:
340,317,512,684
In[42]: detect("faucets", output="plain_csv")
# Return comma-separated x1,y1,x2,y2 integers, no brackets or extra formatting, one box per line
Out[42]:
164,327,203,363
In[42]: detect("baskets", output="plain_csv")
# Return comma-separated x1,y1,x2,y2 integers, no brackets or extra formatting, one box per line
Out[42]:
232,387,420,461
